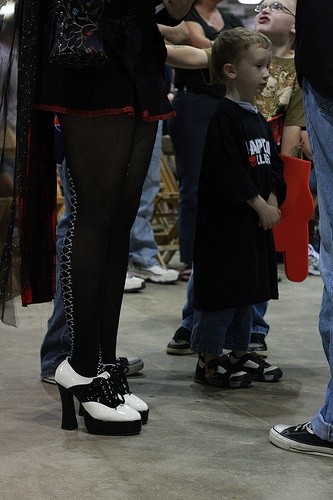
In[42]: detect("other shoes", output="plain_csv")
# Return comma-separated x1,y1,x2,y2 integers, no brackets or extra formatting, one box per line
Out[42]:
124,271,146,292
307,244,320,276
249,332,269,358
127,262,180,282
166,325,193,354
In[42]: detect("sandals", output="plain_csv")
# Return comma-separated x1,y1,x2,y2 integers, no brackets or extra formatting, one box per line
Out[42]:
180,264,192,282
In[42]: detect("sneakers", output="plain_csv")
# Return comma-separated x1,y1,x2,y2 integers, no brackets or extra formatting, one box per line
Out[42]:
268,421,333,457
227,351,283,381
194,352,252,387
44,356,145,385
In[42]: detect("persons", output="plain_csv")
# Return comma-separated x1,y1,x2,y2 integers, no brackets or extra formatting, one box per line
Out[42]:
123,119,180,291
269,0,333,458
299,129,321,276
166,0,242,281
0,0,171,435
155,0,195,94
165,0,303,160
191,27,287,387
166,260,270,357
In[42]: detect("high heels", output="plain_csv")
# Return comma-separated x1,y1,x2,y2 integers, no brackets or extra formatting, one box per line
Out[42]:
103,357,149,423
54,356,141,435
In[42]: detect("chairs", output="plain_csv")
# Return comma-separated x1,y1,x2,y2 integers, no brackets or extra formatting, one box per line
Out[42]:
0,127,181,300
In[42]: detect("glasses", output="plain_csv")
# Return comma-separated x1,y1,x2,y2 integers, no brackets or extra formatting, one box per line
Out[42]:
254,1,295,17
